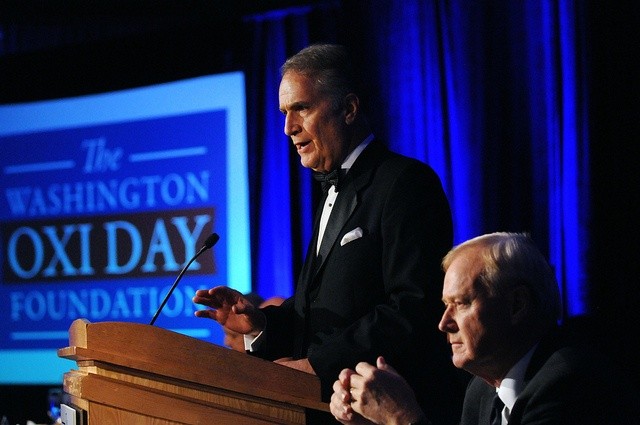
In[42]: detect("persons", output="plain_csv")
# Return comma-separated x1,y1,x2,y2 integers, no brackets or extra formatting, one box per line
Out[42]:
328,232,601,425
193,45,454,425
256,297,316,375
222,293,264,354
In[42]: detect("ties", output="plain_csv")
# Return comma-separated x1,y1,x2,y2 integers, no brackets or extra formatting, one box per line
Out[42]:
491,392,504,424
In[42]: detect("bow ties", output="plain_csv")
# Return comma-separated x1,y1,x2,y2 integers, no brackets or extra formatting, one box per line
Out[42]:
313,170,343,192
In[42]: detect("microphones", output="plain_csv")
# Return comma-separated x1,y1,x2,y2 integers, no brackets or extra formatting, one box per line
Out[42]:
149,232,220,326
